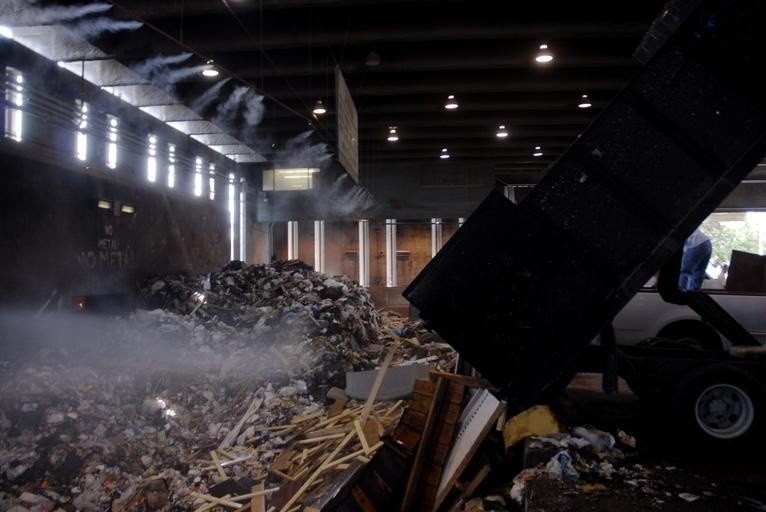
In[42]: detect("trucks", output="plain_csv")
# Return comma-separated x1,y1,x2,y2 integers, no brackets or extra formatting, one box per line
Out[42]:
402,1,766,449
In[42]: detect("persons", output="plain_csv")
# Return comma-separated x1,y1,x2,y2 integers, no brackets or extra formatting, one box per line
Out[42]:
678,229,712,289
719,262,729,288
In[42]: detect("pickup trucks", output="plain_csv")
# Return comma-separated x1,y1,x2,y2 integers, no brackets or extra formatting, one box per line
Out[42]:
589,281,766,372
